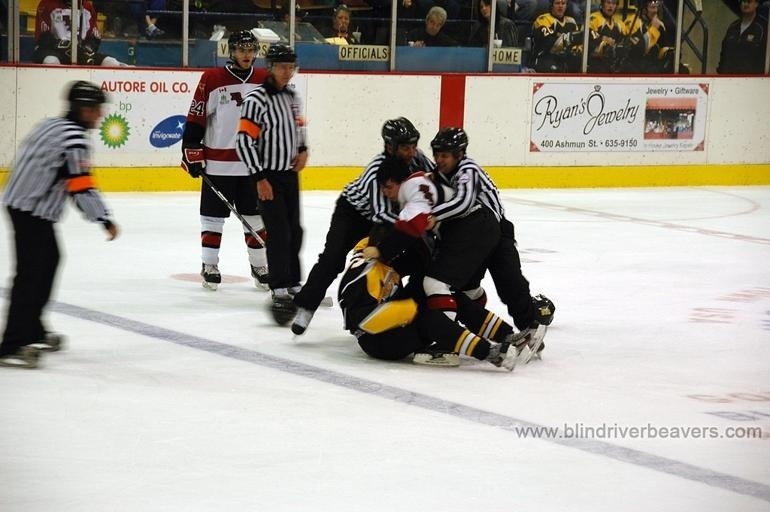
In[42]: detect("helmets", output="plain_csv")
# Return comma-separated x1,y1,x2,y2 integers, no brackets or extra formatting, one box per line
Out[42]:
431,127,468,154
69,81,105,104
266,45,297,62
228,30,259,53
382,117,420,145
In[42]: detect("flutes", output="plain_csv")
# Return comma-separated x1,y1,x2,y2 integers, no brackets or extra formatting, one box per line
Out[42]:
198,170,332,306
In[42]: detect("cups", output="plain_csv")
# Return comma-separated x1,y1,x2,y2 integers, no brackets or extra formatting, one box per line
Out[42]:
128,38,137,66
352,31,361,44
493,39,503,49
338,32,348,43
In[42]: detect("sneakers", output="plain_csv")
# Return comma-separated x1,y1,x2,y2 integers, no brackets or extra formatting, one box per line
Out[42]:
292,310,312,334
489,294,544,367
201,263,221,283
251,265,270,283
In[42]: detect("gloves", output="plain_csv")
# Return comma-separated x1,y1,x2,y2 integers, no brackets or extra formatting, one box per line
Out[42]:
181,148,206,177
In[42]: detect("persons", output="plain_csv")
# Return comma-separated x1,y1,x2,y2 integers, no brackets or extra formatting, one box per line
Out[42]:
716,0,767,74
185,1,555,371
131,0,167,40
33,0,134,67
532,0,585,73
623,0,674,75
589,0,630,72
0,81,121,370
